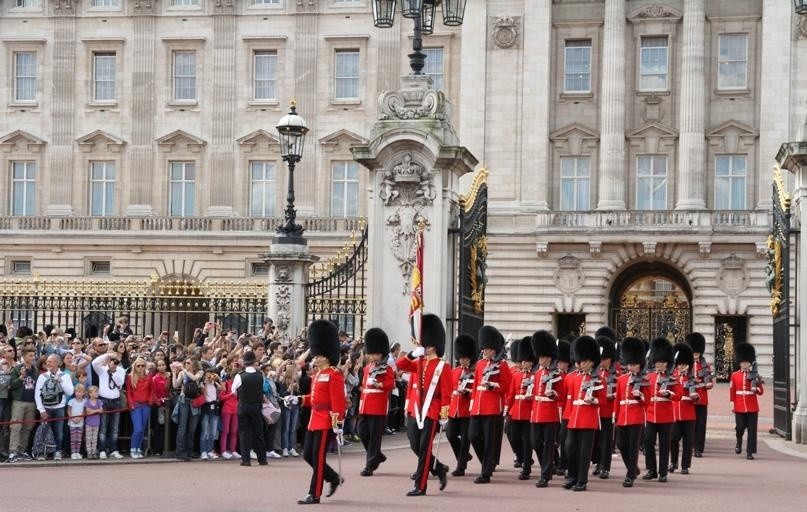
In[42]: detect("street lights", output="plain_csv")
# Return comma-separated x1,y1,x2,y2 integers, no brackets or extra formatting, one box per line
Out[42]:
276,100,308,236
369,0,467,76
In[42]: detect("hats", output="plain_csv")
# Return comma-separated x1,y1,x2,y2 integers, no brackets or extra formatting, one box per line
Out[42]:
364,328,390,356
511,327,705,371
454,334,478,365
308,320,339,365
477,326,504,355
421,315,445,358
735,343,755,364
241,351,256,365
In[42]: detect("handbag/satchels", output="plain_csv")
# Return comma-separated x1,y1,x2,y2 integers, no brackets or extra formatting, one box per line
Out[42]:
33,424,55,454
262,395,281,425
192,393,207,407
183,381,199,397
171,400,180,424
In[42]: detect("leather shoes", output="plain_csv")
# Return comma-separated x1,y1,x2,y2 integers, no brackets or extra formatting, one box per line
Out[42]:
747,454,753,459
514,456,608,490
298,495,319,504
407,488,426,496
695,448,702,457
439,465,448,490
360,467,373,475
736,444,741,453
327,476,344,497
623,444,688,486
451,455,495,483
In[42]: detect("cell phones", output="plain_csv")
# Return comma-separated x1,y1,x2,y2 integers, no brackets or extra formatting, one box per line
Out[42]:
174,330,179,338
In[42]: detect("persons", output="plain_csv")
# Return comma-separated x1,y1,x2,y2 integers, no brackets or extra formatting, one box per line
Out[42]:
1,319,412,468
729,360,764,460
356,326,396,477
398,313,452,496
282,320,343,504
447,325,714,493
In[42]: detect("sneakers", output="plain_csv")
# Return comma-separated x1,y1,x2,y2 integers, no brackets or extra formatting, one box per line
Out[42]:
1,448,144,463
343,433,361,445
176,447,299,466
385,426,392,434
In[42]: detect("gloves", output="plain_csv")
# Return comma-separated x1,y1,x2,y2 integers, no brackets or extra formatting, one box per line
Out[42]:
332,422,345,434
284,394,301,408
412,346,426,358
366,377,378,386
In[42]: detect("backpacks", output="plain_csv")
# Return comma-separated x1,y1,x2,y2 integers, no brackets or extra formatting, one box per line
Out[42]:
39,373,64,406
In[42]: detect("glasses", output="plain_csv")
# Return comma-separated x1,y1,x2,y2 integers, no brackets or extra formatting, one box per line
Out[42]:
25,341,35,344
72,342,79,344
98,343,106,346
8,349,14,352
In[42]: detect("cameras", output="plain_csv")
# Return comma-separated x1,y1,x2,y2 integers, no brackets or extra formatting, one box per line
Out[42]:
246,334,251,338
114,359,119,365
129,343,137,348
25,367,33,376
66,334,71,338
229,330,236,334
235,363,239,367
173,363,180,369
222,332,227,336
208,322,213,327
271,326,275,330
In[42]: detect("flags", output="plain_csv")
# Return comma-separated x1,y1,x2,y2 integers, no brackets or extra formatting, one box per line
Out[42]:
400,224,425,319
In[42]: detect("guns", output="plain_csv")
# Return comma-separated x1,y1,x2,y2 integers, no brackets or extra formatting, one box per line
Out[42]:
746,356,764,391
456,333,716,403
369,342,394,384
